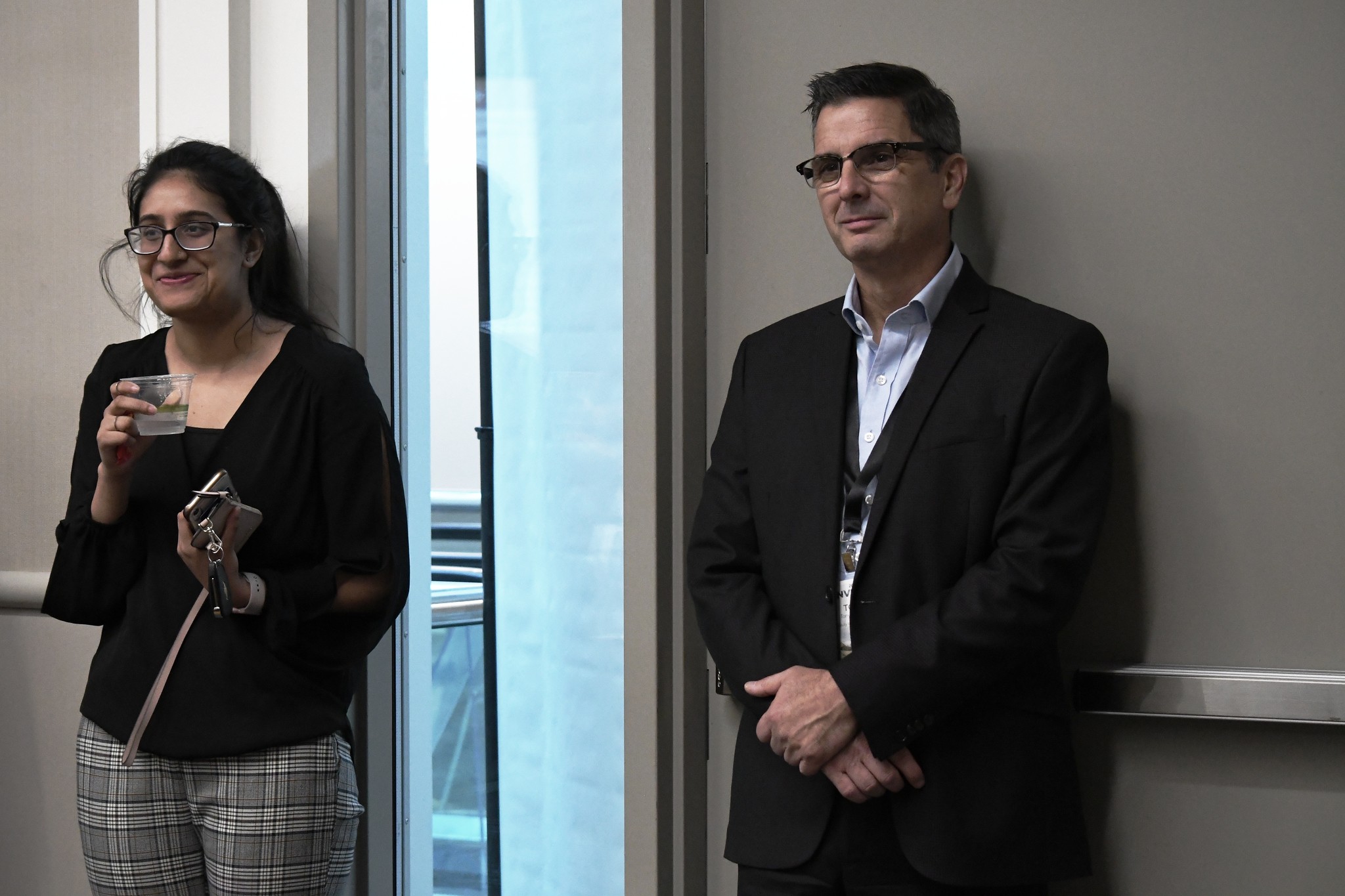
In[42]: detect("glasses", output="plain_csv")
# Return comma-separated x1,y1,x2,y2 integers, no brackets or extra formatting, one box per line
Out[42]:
124,219,245,255
795,141,939,187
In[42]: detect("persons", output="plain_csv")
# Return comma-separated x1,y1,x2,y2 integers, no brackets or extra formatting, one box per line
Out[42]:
38,140,409,896
684,63,1107,896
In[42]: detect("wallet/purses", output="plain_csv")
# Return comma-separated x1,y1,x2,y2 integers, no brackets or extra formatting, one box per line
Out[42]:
190,490,262,556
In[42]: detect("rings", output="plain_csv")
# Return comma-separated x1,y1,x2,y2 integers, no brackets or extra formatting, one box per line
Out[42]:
114,415,119,431
116,381,122,396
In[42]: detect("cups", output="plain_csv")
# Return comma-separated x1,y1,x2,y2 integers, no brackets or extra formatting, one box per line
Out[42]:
120,373,194,436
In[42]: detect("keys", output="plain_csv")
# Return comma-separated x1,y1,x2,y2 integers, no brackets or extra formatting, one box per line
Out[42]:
208,559,232,619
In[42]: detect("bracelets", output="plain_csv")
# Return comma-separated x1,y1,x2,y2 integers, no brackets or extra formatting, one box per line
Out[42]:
231,571,266,616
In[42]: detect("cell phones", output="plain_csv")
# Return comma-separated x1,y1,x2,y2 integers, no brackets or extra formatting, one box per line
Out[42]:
182,469,239,536
